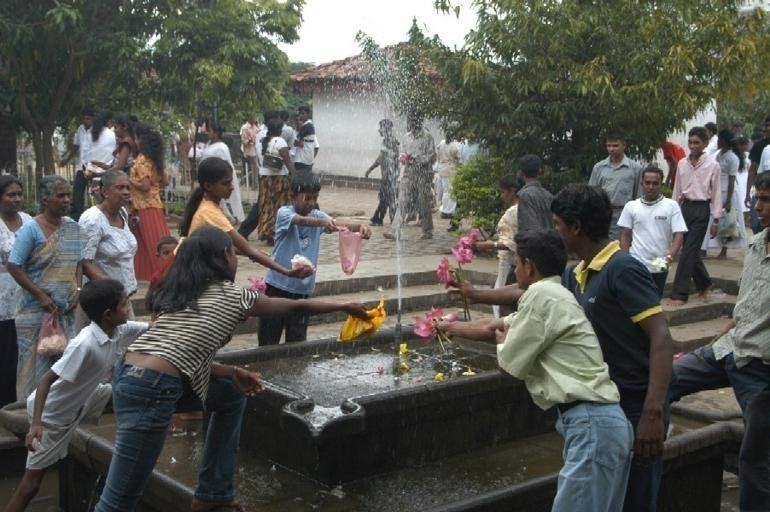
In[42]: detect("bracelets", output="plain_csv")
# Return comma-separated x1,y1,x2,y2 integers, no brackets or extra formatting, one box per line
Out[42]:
75,286,82,293
668,254,673,260
494,241,498,249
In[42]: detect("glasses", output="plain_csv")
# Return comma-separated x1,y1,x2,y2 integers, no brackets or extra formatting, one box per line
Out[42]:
109,183,129,190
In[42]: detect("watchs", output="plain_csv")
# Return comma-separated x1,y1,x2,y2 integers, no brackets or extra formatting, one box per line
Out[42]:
713,222,717,228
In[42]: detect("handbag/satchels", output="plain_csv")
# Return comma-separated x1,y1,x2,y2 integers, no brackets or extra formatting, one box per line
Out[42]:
263,138,284,169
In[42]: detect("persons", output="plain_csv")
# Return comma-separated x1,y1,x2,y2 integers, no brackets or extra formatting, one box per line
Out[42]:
588,128,646,241
617,166,688,302
7,175,85,402
737,138,756,235
703,123,722,159
155,236,178,263
700,127,746,260
257,168,372,347
73,169,138,333
240,112,259,192
60,107,95,222
757,147,765,173
145,158,316,317
671,172,769,511
435,230,634,512
671,126,722,305
383,109,437,240
448,184,673,512
3,279,154,511
447,120,491,231
93,224,377,510
499,156,560,317
289,106,319,211
80,111,116,210
82,114,140,178
258,119,293,245
744,137,770,237
128,123,171,282
366,119,400,226
277,111,294,154
199,125,246,232
0,174,33,407
436,125,462,218
475,174,526,316
239,111,279,242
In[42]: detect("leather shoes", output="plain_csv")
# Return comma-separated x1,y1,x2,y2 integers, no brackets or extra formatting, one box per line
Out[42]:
441,212,454,218
421,232,434,239
383,233,399,241
447,223,457,231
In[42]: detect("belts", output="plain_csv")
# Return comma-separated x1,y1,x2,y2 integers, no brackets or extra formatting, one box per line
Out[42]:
408,163,426,167
610,205,624,210
557,400,585,413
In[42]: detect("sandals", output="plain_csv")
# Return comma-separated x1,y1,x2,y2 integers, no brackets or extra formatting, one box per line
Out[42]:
191,496,245,512
667,299,688,305
697,282,713,296
